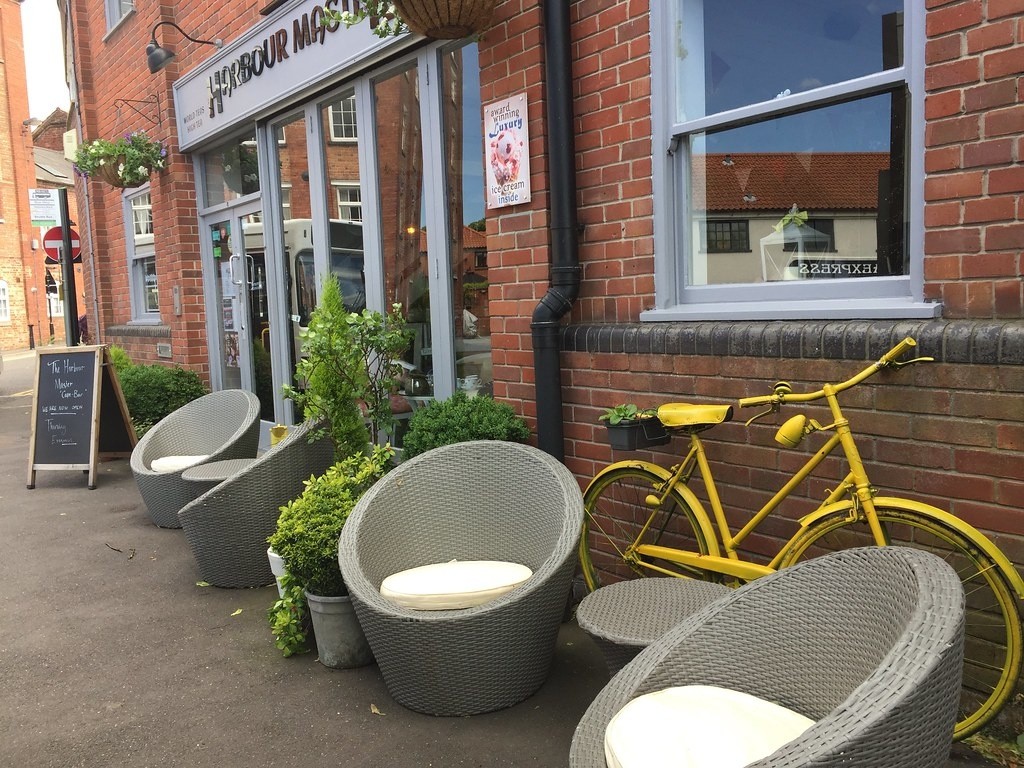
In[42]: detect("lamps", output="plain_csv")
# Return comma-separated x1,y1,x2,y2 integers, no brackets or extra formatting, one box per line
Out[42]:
145,21,223,74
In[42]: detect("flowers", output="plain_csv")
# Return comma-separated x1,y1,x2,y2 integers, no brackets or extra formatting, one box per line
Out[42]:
66,128,170,187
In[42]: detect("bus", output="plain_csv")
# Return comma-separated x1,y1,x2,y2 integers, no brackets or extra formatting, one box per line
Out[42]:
242,219,363,390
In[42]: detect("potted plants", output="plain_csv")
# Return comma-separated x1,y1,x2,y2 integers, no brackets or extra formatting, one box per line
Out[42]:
266,442,395,670
598,403,672,452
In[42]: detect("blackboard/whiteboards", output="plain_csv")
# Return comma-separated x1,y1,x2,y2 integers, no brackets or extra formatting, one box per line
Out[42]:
28,344,138,471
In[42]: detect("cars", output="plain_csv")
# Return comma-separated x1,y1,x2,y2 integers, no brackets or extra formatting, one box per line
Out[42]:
431,359,491,405
297,327,433,440
419,335,491,370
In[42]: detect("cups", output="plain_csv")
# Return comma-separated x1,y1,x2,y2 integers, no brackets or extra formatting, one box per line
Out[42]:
456,375,482,390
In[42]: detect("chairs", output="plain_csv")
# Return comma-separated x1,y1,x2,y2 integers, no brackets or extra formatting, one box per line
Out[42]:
569,547,968,768
177,414,336,589
338,440,584,717
129,388,261,529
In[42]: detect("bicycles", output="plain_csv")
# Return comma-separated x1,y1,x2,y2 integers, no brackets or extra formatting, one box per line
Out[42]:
578,333,1024,750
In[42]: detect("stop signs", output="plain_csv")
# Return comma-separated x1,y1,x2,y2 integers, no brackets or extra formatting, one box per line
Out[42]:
42,226,82,261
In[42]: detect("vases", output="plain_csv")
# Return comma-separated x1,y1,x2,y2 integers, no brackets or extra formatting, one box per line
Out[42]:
267,544,291,600
95,152,153,188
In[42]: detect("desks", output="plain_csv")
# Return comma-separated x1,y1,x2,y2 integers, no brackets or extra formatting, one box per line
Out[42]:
180,458,258,482
576,577,737,679
